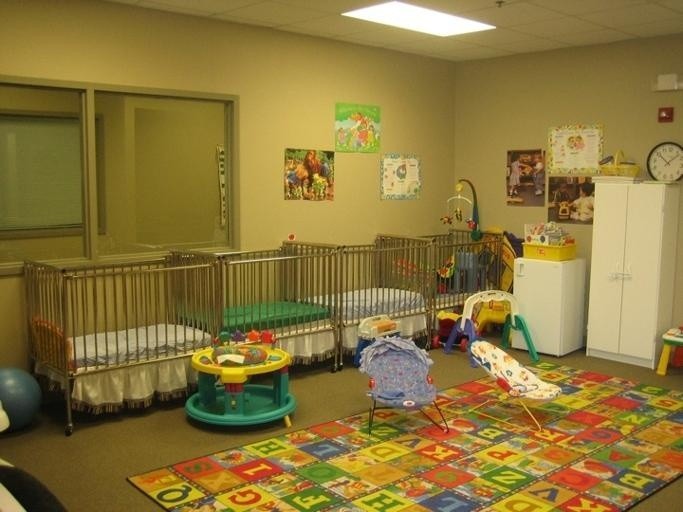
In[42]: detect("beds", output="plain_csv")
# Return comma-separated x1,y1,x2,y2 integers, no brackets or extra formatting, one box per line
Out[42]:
21,252,219,435
166,236,340,373
283,236,435,371
377,233,504,347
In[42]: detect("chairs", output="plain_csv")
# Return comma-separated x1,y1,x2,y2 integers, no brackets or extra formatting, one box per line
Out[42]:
467,334,570,432
358,336,450,433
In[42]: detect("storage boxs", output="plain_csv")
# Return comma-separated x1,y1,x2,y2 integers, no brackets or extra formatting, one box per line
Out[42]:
521,241,576,261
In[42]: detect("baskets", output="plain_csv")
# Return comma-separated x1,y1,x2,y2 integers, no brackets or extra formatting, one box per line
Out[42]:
602,150,642,178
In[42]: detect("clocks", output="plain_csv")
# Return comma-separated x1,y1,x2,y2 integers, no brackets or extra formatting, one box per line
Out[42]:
647,141,683,182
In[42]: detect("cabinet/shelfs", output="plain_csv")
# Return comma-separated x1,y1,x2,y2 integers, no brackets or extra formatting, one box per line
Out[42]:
512,257,586,358
586,175,683,370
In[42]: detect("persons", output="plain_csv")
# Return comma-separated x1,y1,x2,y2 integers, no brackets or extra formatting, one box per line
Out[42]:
554,182,575,215
337,112,376,147
567,134,585,150
300,150,328,198
531,153,544,196
509,152,528,197
568,182,594,222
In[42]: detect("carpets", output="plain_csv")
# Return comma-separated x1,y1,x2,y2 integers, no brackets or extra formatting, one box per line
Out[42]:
124,362,683,511
